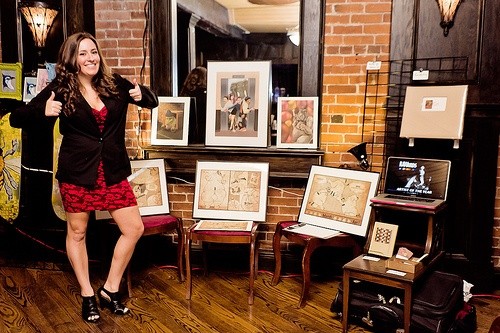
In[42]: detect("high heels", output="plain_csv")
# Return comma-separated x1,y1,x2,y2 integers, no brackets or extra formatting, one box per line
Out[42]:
97,286,130,315
81,293,100,323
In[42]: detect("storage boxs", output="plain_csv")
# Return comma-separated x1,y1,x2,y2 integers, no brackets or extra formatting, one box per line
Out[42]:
386,255,424,273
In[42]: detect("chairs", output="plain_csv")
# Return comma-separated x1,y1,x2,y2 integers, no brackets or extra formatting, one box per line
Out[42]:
272,220,360,307
343,203,435,333
100,214,184,298
185,219,260,304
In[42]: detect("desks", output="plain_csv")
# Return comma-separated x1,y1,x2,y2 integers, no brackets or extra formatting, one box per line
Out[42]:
372,198,447,262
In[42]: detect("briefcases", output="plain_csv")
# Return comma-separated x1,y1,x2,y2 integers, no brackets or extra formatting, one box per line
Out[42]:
329,267,479,333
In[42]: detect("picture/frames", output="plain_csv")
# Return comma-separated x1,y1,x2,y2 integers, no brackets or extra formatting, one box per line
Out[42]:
95,159,171,220
151,95,190,146
206,60,270,147
276,97,318,149
299,164,380,236
192,161,270,222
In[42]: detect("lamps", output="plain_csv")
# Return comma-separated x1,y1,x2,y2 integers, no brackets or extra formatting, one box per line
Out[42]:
284,28,300,47
434,0,465,37
18,2,59,55
347,141,369,170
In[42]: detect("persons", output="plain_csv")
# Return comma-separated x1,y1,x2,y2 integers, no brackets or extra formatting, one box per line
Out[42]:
180,67,207,145
414,166,429,191
279,88,289,97
223,93,254,132
9,33,159,322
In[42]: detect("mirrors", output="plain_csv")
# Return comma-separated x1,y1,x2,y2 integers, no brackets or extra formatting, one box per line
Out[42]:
148,0,324,147
